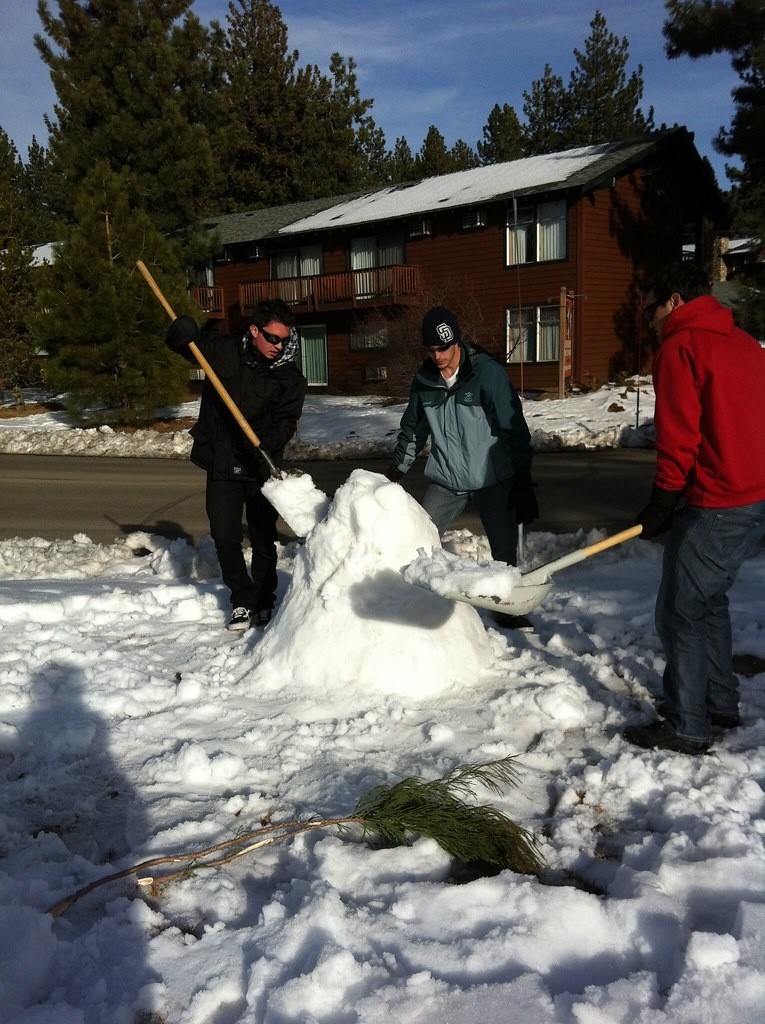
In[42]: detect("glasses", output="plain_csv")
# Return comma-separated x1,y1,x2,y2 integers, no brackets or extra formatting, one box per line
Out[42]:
641,301,659,324
255,327,291,348
424,344,452,353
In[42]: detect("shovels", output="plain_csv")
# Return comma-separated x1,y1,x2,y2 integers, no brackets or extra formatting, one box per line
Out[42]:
136,258,332,537
399,524,641,617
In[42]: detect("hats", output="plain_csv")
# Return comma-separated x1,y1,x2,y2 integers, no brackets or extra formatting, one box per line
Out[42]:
421,306,463,347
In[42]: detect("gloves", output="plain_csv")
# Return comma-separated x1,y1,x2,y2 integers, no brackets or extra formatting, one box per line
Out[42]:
171,313,198,346
253,431,274,462
635,481,688,540
506,471,540,524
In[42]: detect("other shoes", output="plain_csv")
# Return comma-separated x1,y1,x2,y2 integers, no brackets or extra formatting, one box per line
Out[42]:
657,702,740,729
624,719,711,755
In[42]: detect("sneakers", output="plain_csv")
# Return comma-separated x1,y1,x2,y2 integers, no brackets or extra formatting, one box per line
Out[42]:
256,606,273,626
227,607,254,630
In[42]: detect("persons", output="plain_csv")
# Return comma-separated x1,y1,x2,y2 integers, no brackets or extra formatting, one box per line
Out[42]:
387,303,539,630
166,298,308,632
628,262,765,752
